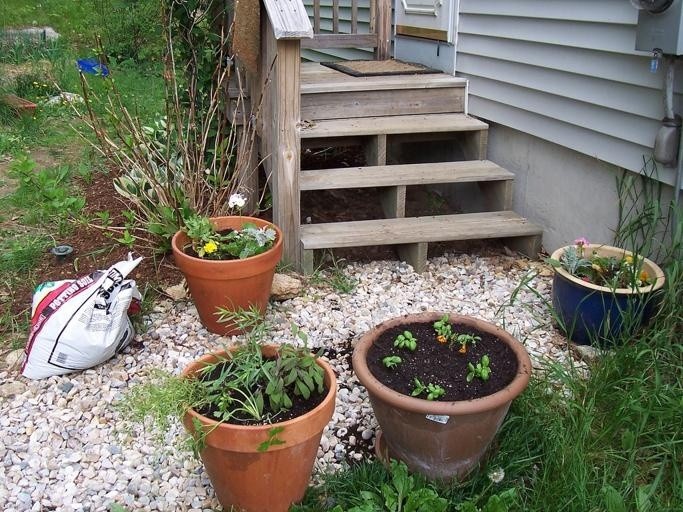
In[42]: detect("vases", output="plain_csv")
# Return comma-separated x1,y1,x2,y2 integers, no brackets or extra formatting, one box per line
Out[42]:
548,242,664,345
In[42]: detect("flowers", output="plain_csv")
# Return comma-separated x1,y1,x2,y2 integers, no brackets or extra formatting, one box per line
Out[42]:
572,237,648,285
184,192,271,256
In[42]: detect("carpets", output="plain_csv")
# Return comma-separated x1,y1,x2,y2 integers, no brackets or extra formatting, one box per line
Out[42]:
319,57,442,76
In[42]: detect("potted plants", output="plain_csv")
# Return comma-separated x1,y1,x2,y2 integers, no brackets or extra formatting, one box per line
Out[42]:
351,311,532,479
172,216,284,335
115,303,338,512
